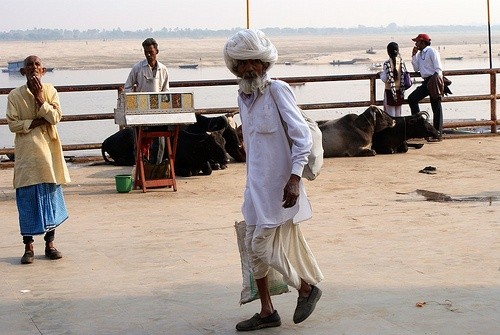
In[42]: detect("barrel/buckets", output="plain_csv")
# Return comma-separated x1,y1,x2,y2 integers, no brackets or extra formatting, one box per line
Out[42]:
115,174,132,193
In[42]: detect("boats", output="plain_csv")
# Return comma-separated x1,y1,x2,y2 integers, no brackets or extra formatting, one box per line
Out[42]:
329,58,356,64
369,61,383,71
2,60,54,73
179,65,198,69
366,48,377,54
445,56,464,60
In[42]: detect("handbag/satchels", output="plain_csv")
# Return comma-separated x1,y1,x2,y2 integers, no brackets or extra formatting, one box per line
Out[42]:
233,221,291,304
269,80,325,181
404,72,411,90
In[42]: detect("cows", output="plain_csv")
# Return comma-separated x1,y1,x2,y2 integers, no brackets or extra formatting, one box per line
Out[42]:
102,105,440,177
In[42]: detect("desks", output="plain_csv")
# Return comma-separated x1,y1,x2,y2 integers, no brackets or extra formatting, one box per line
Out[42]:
119,124,180,193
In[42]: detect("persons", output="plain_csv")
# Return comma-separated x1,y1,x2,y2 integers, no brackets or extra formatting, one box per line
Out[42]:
223,29,323,332
6,55,71,264
408,33,443,142
377,42,407,117
124,38,169,165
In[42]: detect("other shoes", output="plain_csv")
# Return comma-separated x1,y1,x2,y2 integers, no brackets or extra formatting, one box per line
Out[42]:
45,245,62,259
426,136,439,142
21,249,34,263
236,310,281,331
293,284,322,324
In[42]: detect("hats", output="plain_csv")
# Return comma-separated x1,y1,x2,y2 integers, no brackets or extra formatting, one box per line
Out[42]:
224,28,278,76
412,34,431,43
